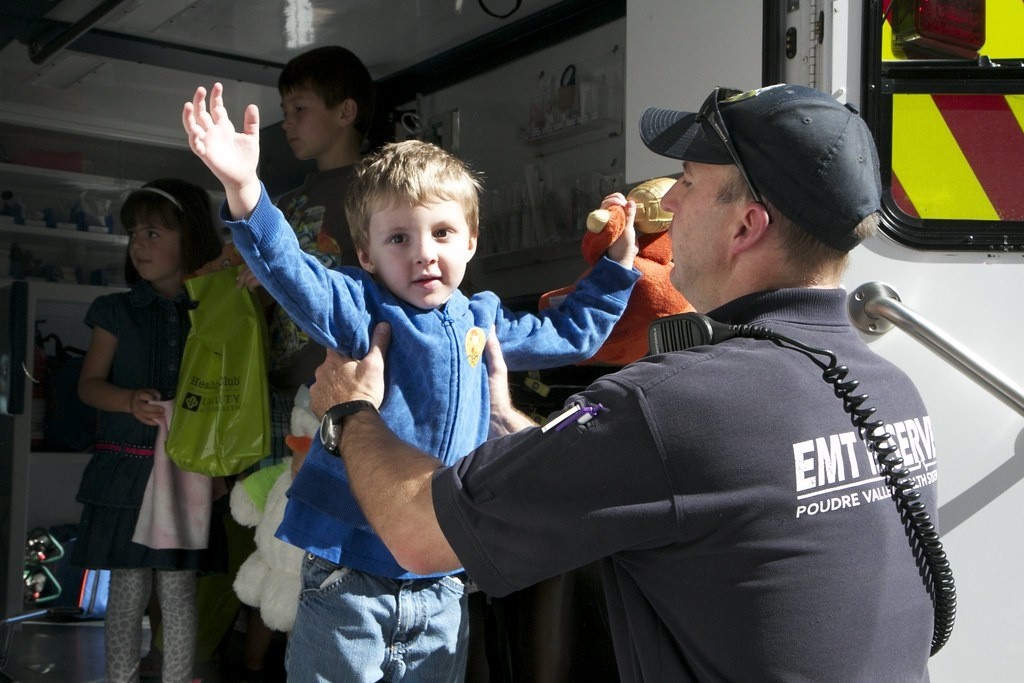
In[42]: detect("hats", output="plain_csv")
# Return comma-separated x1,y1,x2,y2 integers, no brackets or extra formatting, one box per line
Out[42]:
638,83,881,252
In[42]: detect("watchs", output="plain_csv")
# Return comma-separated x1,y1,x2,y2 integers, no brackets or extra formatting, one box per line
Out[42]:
319,399,379,456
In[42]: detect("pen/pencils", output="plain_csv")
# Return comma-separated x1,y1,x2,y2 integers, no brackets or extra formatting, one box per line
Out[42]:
541,404,580,433
555,402,603,432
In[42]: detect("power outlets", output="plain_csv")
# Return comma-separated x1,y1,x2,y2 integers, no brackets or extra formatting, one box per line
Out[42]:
464,104,484,133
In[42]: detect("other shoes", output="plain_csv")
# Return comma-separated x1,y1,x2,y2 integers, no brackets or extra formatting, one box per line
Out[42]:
139,651,164,676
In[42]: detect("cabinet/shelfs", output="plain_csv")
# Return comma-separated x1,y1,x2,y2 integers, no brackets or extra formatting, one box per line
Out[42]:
0,119,226,625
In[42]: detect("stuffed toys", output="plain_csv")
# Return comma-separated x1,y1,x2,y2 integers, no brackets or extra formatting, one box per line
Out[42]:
539,197,696,367
228,391,318,632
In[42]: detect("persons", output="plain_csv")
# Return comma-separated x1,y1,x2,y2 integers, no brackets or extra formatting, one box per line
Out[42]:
304,81,939,683
174,77,639,683
233,41,378,681
75,176,235,681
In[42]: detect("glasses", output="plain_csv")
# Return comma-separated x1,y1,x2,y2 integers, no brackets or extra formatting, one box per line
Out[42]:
694,86,773,224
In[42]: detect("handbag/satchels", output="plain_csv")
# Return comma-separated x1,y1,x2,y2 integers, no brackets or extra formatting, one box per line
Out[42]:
164,261,272,476
